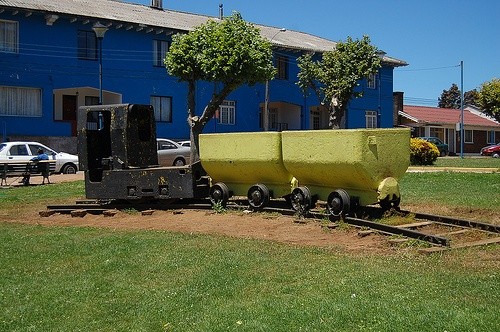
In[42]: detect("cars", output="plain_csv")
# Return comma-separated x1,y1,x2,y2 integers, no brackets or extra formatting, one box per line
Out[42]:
418,137,448,156
480,143,500,158
155,138,193,167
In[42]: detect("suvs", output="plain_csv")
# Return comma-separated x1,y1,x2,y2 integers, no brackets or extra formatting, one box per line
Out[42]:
0,142,79,175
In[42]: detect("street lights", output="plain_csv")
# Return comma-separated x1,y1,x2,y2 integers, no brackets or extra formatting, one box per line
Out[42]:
262,29,287,131
89,23,107,103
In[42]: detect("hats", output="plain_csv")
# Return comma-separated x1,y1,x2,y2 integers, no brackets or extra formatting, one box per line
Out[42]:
37,148,43,154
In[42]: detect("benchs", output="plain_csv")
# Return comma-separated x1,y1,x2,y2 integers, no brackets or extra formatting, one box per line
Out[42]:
0,160,57,189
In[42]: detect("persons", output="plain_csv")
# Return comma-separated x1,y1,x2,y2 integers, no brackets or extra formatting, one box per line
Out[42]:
18,147,50,185
